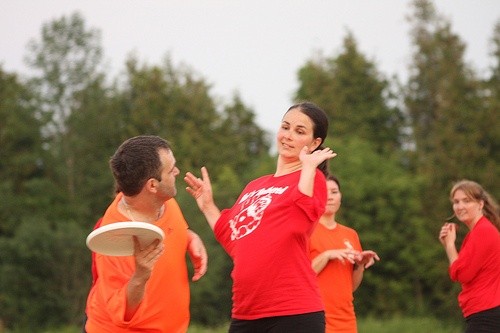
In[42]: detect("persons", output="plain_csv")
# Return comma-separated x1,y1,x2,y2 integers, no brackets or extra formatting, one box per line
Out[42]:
307,173,380,333
84,134,208,333
439,180,500,332
184,101,337,332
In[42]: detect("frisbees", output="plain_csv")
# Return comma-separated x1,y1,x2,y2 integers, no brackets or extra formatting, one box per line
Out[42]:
86,221,165,257
